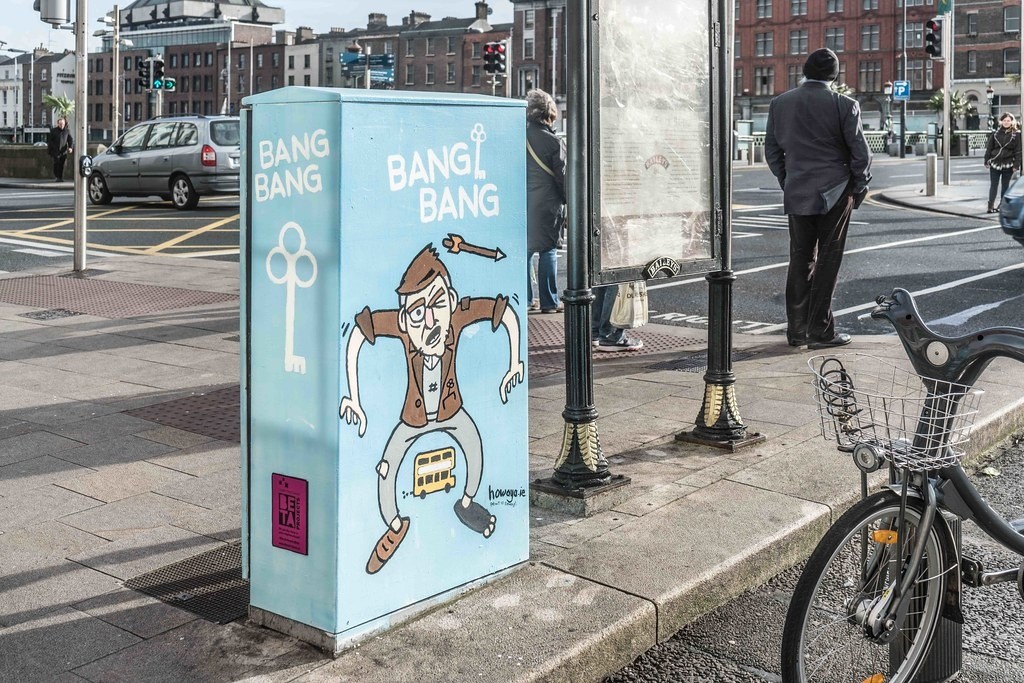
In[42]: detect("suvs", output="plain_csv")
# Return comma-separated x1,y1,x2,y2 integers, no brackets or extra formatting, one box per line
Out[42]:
89,114,240,212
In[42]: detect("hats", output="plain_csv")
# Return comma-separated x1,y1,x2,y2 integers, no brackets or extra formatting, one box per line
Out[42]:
802,48,839,81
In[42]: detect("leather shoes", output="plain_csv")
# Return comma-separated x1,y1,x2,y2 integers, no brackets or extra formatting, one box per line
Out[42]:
808,333,853,348
788,338,807,346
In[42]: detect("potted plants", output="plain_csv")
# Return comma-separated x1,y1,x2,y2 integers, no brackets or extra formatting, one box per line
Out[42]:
926,87,974,156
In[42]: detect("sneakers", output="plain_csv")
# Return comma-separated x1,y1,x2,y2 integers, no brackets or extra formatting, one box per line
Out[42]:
592,337,599,346
599,329,643,351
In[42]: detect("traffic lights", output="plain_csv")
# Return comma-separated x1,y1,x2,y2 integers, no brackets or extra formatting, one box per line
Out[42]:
137,60,152,93
483,42,499,76
925,18,950,65
164,78,177,92
151,59,165,91
494,40,509,76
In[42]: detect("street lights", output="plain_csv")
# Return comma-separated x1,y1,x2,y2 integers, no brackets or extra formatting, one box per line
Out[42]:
346,40,363,89
985,84,999,132
94,4,135,145
884,80,895,133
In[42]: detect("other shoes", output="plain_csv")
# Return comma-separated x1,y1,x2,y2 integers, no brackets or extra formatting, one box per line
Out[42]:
997,204,1001,212
59,178,63,182
527,299,541,313
987,208,993,213
55,177,59,182
541,303,565,313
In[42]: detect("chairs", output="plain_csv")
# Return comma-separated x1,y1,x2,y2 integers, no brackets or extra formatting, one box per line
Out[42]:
224,130,240,141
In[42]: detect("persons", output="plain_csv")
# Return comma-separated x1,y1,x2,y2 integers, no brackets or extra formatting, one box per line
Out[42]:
526,89,567,314
984,113,1022,212
48,119,73,182
765,47,873,349
591,285,643,351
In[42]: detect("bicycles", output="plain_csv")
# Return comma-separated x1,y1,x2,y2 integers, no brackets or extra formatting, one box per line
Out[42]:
780,286,1022,683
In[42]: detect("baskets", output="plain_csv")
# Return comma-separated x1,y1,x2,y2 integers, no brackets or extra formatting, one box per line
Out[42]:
808,352,986,473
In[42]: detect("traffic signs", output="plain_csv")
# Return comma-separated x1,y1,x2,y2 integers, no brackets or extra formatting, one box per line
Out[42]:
370,54,396,83
339,52,366,77
894,79,912,103
